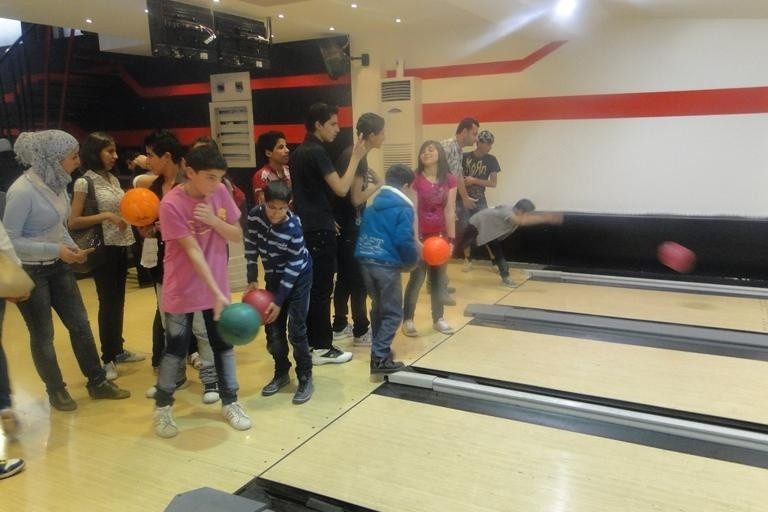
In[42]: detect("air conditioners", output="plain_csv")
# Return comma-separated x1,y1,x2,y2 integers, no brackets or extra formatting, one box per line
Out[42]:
378,76,422,185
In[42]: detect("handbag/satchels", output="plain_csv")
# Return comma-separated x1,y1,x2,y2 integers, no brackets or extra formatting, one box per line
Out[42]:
71,233,99,275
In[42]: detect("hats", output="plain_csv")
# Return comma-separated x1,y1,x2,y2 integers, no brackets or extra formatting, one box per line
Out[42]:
479,131,494,143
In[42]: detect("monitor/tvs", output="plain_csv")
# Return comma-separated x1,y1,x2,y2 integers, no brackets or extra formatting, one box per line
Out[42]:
21,22,102,78
147,0,218,65
213,11,271,69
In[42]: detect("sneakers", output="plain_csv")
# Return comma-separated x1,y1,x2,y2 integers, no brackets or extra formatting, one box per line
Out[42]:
488,260,518,288
442,287,455,307
262,371,290,395
186,353,202,368
293,375,313,404
402,319,418,338
50,387,78,411
432,317,455,333
88,350,145,400
369,351,405,374
462,257,472,272
0,458,25,479
154,405,178,438
203,382,219,403
146,379,187,398
221,401,252,431
308,322,372,365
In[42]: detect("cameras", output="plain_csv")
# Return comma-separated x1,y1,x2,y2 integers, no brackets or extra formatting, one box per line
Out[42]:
50,257,72,276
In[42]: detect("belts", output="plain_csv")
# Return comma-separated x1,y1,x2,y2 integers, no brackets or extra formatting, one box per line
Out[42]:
20,257,59,265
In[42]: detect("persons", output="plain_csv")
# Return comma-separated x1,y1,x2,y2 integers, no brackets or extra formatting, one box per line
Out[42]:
242,100,386,404
426,117,500,305
2,130,131,411
453,199,562,288
352,164,418,374
402,141,457,337
0,223,35,480
127,130,253,438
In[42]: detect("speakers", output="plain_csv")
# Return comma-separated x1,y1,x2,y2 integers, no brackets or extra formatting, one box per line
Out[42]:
319,43,351,80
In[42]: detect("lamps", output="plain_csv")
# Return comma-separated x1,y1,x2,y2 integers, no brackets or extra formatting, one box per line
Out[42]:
319,36,369,82
